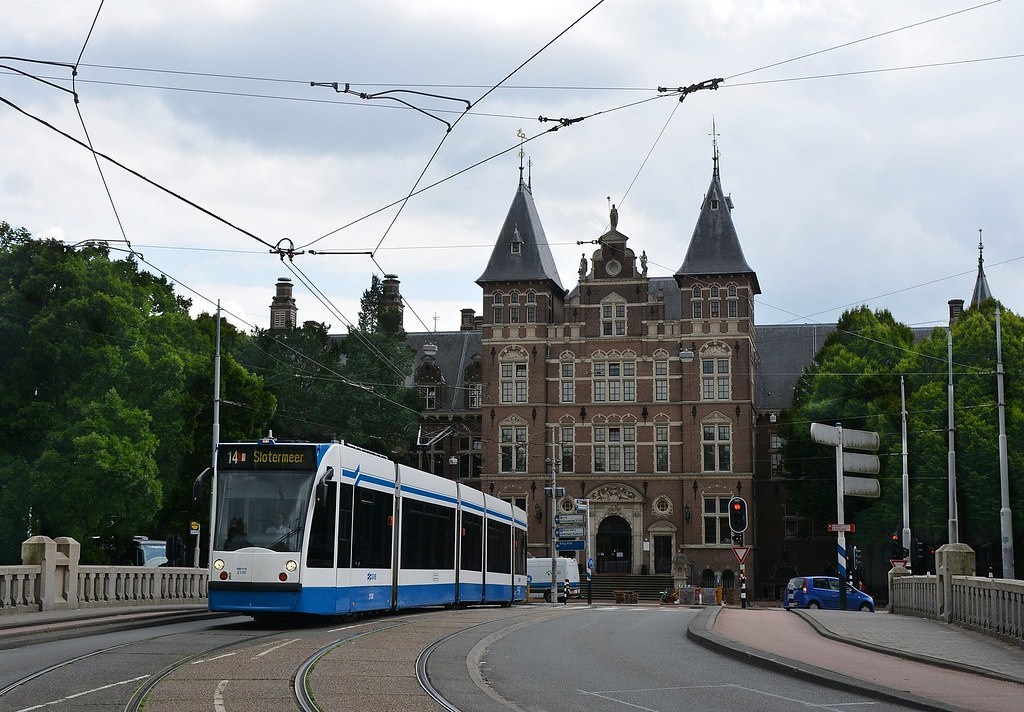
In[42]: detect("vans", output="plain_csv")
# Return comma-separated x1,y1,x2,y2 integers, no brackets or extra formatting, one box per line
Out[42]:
527,557,581,604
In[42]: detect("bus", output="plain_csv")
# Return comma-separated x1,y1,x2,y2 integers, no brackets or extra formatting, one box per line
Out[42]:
191,422,529,626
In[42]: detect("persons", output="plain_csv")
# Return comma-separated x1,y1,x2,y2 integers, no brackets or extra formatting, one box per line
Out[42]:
578,253,588,280
858,580,867,594
609,204,618,231
264,511,294,533
640,250,648,279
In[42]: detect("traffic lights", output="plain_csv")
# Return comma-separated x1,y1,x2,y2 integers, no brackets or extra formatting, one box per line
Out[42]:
853,546,863,570
912,538,924,563
891,532,901,558
928,546,935,559
846,554,850,566
727,497,748,534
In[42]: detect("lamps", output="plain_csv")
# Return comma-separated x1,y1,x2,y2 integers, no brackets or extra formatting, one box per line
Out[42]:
581,481,586,497
735,404,741,424
691,405,697,425
643,480,649,499
585,286,592,305
530,480,537,500
531,407,537,427
534,504,543,525
489,480,495,495
579,406,587,425
636,284,641,301
641,405,649,425
692,480,699,501
691,340,697,360
734,341,740,360
678,342,684,352
649,307,656,320
683,504,691,524
490,408,496,427
532,346,538,365
572,308,578,321
490,347,496,366
736,481,742,496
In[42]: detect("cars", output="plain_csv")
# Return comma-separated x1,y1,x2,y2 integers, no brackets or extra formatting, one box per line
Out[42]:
782,575,875,614
